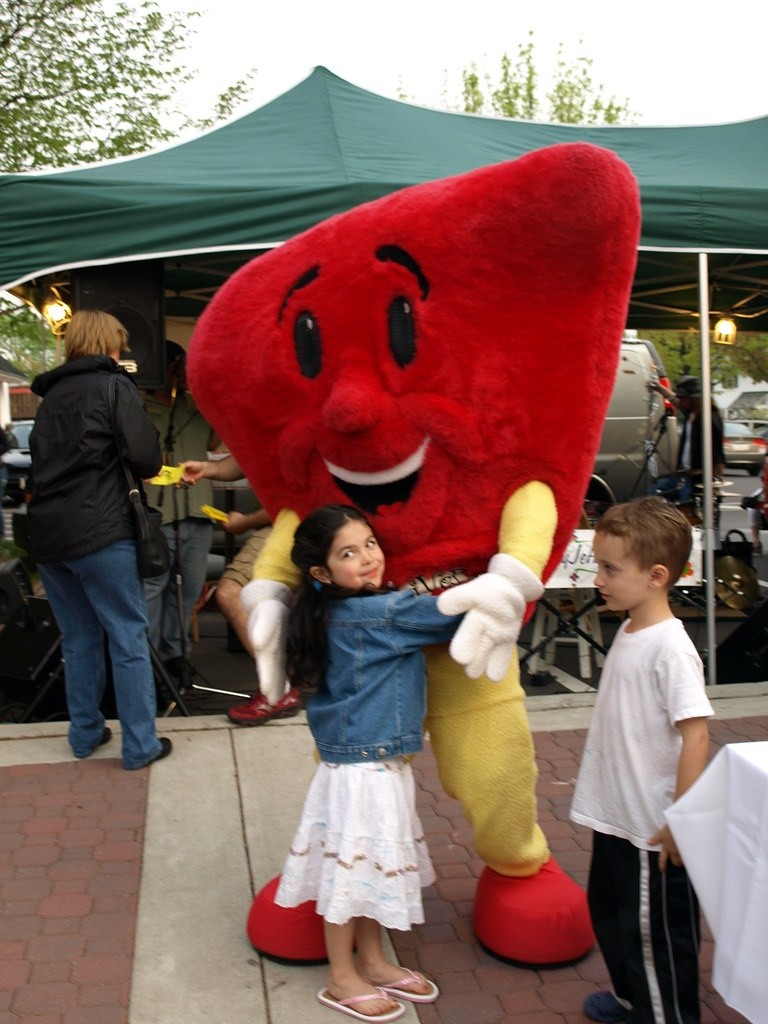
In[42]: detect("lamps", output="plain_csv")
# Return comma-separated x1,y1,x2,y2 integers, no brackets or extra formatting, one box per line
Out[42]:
41,294,73,335
713,306,738,345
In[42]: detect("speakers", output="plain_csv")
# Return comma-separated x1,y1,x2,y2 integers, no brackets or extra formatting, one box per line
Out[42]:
69,262,170,395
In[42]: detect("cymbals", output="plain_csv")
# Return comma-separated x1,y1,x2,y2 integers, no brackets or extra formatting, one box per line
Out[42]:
694,489,742,497
695,481,735,489
714,555,759,609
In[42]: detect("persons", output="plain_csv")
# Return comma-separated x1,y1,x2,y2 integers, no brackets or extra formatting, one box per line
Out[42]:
747,471,768,553
273,502,467,1023
647,376,725,530
0,310,301,770
569,496,715,1024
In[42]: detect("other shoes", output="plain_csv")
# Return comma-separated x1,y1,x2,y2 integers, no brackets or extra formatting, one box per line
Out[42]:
582,992,640,1024
146,736,172,766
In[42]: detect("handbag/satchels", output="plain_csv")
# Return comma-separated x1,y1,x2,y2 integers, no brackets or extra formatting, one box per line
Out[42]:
136,507,172,578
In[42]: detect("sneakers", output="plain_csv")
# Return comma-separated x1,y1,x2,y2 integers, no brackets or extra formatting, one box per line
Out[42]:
225,689,300,725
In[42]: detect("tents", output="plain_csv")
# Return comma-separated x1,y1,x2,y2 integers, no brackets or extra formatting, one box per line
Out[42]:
0,66,768,687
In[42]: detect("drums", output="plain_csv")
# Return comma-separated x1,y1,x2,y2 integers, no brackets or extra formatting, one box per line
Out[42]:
583,473,618,527
647,468,703,525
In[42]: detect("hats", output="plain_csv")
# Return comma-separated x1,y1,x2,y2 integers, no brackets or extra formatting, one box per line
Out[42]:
672,375,702,397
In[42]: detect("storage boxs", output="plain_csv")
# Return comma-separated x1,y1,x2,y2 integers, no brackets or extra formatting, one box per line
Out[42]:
544,526,703,586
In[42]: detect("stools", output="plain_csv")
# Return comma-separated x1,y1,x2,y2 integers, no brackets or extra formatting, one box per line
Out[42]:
528,587,605,678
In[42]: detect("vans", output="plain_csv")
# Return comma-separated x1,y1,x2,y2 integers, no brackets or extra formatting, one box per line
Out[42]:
572,337,680,531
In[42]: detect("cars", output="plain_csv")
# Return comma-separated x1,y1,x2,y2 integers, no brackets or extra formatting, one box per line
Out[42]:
0,421,37,501
719,422,767,476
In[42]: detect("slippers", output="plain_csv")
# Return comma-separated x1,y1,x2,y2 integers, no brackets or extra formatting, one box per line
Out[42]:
374,958,441,1004
75,726,111,758
317,984,406,1023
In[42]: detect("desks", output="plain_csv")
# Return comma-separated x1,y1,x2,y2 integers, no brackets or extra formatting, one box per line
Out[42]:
518,585,608,666
662,739,768,1024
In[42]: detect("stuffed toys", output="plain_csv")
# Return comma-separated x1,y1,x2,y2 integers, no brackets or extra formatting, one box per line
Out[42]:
184,140,641,969
